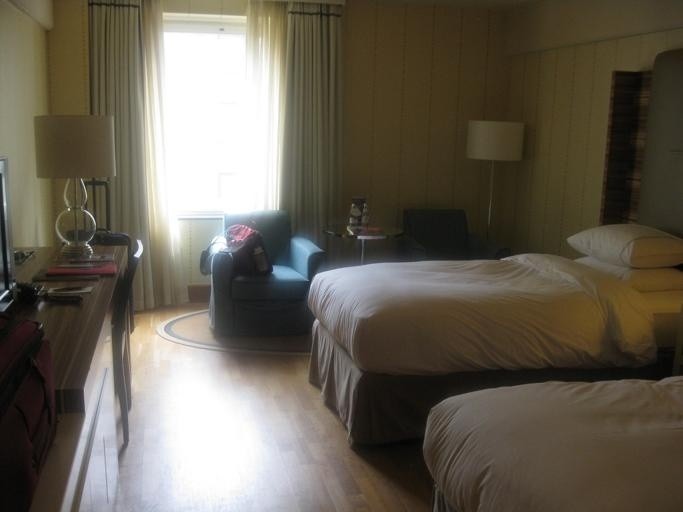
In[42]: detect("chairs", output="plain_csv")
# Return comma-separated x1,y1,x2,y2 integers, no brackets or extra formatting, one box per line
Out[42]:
398,206,504,263
110,239,144,443
207,210,326,338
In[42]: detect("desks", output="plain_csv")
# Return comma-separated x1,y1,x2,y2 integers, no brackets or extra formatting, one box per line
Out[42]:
322,222,403,265
0,247,130,512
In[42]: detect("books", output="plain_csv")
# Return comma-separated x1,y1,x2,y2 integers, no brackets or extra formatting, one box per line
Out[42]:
347,225,387,241
32,253,116,282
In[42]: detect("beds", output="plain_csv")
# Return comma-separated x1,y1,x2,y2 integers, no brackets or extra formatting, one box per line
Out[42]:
306,253,683,448
422,376,683,512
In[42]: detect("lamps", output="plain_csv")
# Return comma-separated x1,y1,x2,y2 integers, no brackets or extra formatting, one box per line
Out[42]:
33,112,116,259
465,119,526,240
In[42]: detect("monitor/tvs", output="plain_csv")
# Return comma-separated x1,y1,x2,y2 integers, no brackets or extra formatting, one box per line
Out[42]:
0,157,19,314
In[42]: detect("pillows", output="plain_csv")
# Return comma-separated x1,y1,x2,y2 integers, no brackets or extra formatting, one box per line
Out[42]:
572,257,683,292
567,224,683,269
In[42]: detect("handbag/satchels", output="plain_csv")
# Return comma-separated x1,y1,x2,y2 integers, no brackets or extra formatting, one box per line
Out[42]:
67,226,132,263
227,224,273,275
